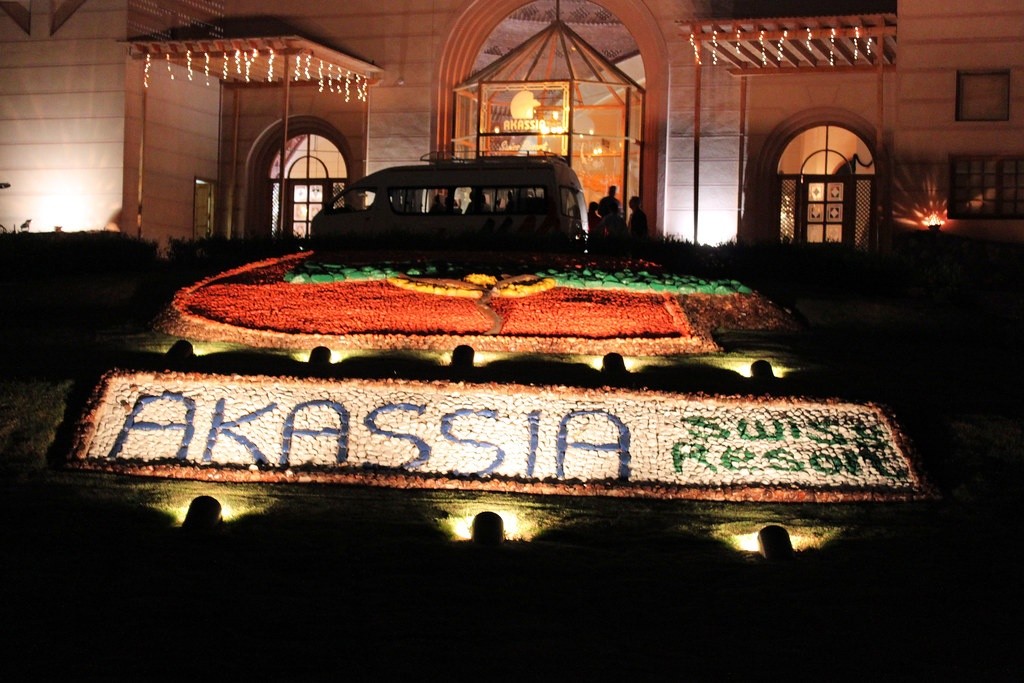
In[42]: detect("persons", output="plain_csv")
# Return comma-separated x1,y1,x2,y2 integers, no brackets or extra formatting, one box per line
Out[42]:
627,195,647,243
503,190,513,211
426,194,444,213
443,195,462,213
592,200,628,239
463,190,486,214
597,185,620,217
586,201,605,236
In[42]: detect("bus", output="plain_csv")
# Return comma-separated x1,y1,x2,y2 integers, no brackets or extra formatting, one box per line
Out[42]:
311,156,581,248
